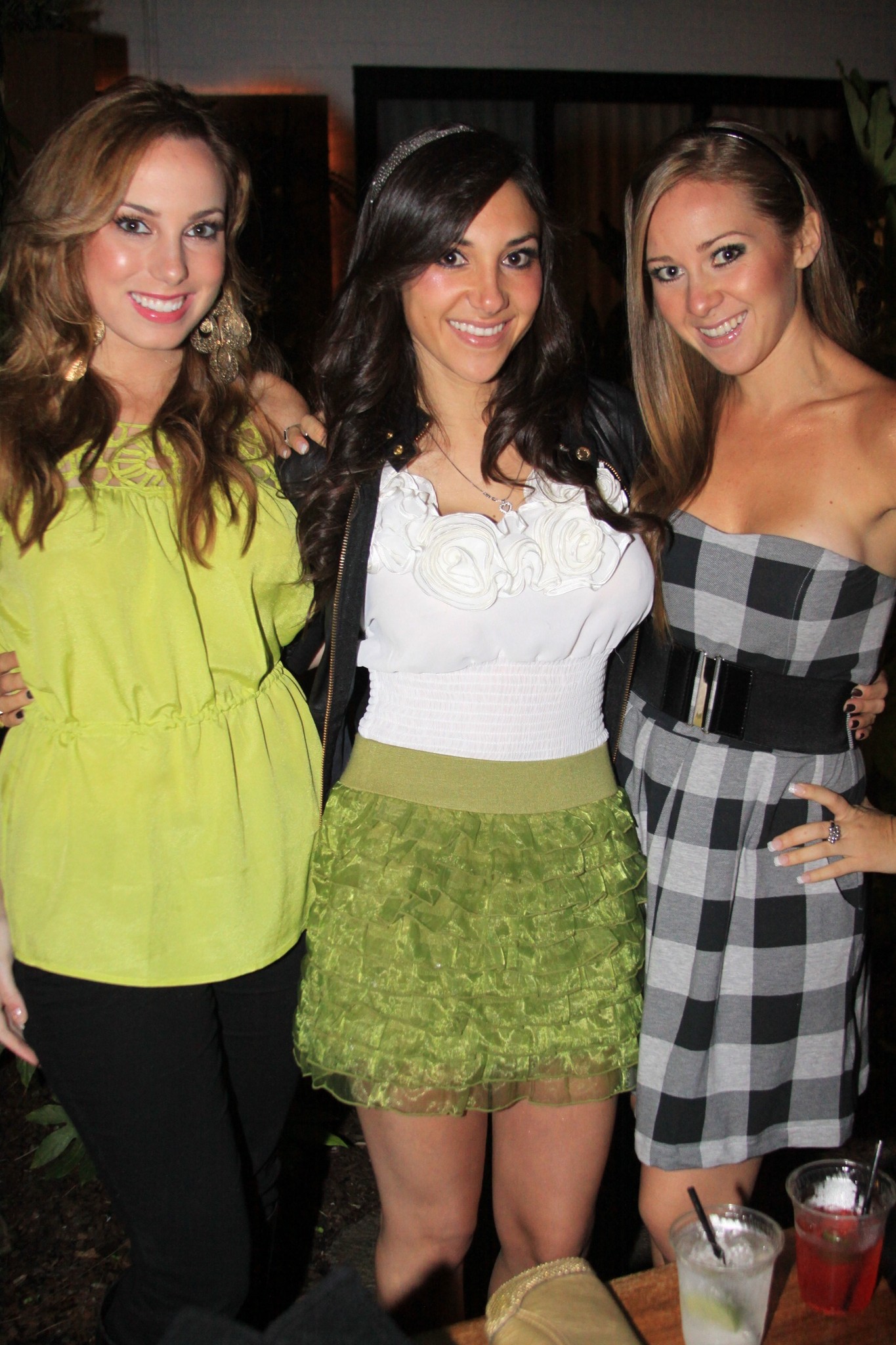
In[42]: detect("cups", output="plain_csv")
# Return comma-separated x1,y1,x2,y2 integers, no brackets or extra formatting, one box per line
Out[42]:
784,1160,896,1316
670,1204,785,1344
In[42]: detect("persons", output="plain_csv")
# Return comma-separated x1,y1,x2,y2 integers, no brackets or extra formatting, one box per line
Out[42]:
2,72,323,1345
273,122,896,1266
0,125,889,1319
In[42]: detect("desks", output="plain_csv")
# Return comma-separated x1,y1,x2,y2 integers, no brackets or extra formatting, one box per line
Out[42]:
416,1227,896,1345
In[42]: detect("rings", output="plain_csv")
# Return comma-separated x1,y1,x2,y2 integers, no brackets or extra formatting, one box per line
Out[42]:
283,424,298,444
828,819,840,844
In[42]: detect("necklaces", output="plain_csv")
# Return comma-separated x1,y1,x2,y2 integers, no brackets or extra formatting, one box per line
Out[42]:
426,429,525,513
